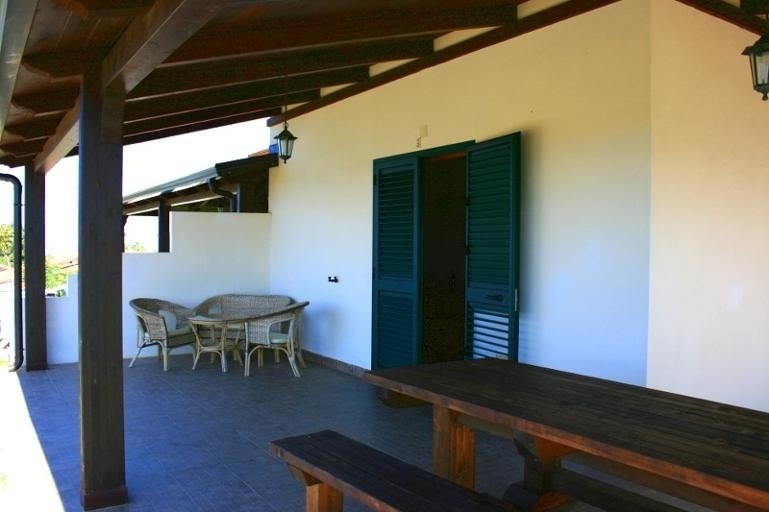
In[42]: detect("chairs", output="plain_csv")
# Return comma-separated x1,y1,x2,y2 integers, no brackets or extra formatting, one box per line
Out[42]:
128,298,197,371
244,301,309,377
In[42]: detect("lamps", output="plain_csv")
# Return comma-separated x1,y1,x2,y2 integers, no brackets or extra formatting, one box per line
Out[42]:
741,0,769,100
273,76,298,164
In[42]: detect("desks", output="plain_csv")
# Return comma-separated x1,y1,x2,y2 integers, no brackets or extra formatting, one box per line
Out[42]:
363,358,769,512
184,313,250,373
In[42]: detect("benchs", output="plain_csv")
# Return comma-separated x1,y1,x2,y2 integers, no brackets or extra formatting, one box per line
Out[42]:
270,403,497,512
193,293,292,361
514,438,769,512
